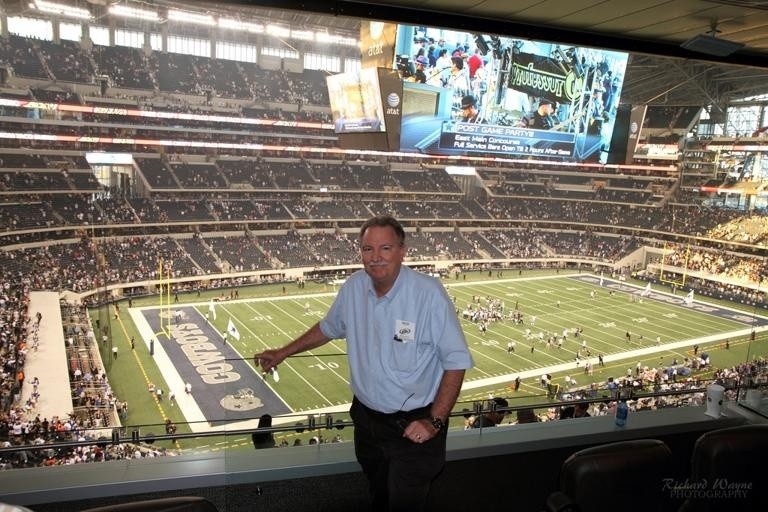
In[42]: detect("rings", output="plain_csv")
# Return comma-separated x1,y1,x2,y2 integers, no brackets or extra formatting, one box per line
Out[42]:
416,434,421,439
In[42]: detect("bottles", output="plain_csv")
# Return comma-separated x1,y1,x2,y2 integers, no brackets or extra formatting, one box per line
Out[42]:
616,399,628,426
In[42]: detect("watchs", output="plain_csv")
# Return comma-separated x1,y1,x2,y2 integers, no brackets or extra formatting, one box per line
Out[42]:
426,414,445,429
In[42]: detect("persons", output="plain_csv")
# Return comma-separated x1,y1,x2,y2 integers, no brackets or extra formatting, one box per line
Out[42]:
0,32,768,470
254,218,473,512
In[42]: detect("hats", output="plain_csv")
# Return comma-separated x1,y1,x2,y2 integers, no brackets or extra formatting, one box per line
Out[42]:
491,398,512,414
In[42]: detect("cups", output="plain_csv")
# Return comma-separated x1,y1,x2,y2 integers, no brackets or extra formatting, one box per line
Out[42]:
704,385,724,419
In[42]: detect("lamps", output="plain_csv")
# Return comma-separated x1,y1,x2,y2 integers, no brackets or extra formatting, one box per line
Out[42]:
679,24,745,57
512,40,525,55
596,62,609,76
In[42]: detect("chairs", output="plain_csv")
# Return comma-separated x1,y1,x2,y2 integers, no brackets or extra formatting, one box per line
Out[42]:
546,422,767,512
